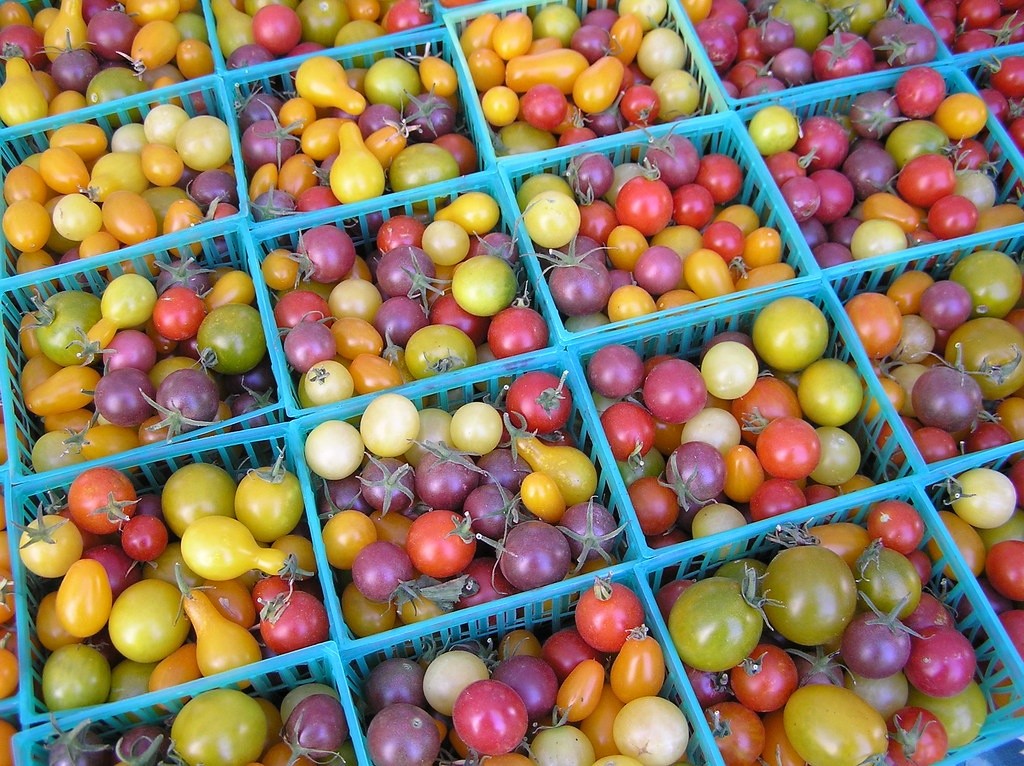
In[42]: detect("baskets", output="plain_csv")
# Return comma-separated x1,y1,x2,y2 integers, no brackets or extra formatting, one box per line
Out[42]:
1,0,1023,764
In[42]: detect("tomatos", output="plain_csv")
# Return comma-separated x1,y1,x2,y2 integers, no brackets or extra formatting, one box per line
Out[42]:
0,0,1024,766
